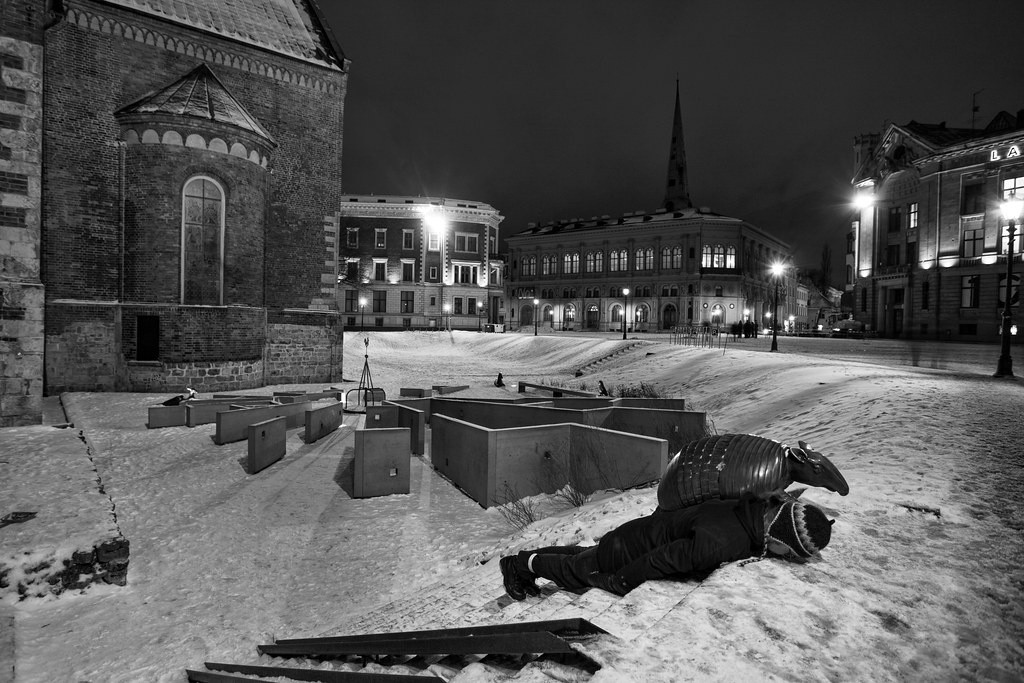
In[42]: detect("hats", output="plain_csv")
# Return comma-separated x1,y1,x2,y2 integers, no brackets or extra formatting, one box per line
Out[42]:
737,501,836,567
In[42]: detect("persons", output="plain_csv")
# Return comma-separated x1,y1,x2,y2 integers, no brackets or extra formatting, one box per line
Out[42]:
499,498,836,600
737,320,758,338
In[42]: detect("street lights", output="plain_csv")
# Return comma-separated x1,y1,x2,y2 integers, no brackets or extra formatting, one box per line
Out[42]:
991,199,1021,381
360,298,365,330
771,262,784,352
477,301,482,333
444,303,451,331
623,288,629,340
534,299,539,335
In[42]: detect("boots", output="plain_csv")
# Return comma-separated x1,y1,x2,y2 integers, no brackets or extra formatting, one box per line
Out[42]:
500,551,541,602
